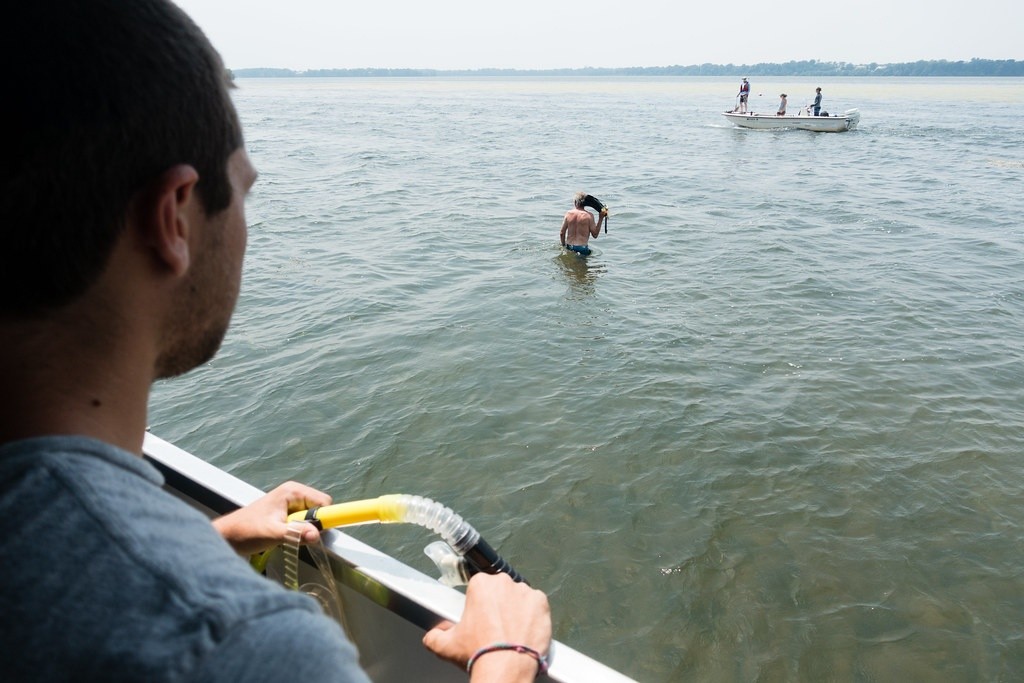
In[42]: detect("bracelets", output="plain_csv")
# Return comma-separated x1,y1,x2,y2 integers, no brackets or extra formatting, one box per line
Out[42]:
466,642,548,680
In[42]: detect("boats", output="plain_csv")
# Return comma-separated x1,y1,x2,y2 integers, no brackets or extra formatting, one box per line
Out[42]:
721,107,861,133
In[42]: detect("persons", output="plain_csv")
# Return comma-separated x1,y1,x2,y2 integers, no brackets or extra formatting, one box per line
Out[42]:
736,77,750,113
0,0,553,683
810,87,822,116
559,191,608,255
777,94,787,115
